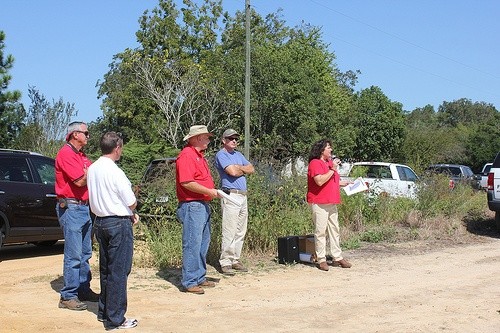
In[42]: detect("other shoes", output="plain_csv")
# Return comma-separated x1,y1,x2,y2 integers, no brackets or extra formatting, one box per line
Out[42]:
332,259,351,268
317,261,329,271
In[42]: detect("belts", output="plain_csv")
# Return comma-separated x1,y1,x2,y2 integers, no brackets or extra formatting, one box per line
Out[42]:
228,189,248,195
112,216,131,219
67,200,89,205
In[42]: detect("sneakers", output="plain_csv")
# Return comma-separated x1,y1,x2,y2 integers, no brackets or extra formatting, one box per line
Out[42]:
58,298,87,311
78,287,100,301
182,280,215,294
98,318,138,331
221,263,248,275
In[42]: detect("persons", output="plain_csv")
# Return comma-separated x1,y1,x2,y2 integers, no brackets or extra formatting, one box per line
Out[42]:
215,129,254,275
175,124,225,294
87,132,139,330
55,121,101,310
306,139,354,271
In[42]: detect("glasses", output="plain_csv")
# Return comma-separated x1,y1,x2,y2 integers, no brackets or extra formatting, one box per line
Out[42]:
73,130,88,136
227,137,238,141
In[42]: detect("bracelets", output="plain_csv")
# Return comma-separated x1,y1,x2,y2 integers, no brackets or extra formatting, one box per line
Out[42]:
330,168,335,172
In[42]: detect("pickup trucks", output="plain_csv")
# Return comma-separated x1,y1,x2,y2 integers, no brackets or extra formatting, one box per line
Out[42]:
339,162,429,203
426,164,482,191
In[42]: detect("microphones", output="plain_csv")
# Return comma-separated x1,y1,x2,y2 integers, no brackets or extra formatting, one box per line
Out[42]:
332,155,342,166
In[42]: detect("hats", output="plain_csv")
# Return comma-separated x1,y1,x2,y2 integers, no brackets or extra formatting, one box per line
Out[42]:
223,128,240,137
183,125,209,141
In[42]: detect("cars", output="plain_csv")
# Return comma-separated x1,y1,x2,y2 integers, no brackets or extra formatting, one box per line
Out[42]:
0,149,98,261
487,151,500,232
478,163,494,190
135,157,179,231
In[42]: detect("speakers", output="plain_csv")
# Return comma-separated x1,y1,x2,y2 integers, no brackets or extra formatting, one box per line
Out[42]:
278,236,300,264
